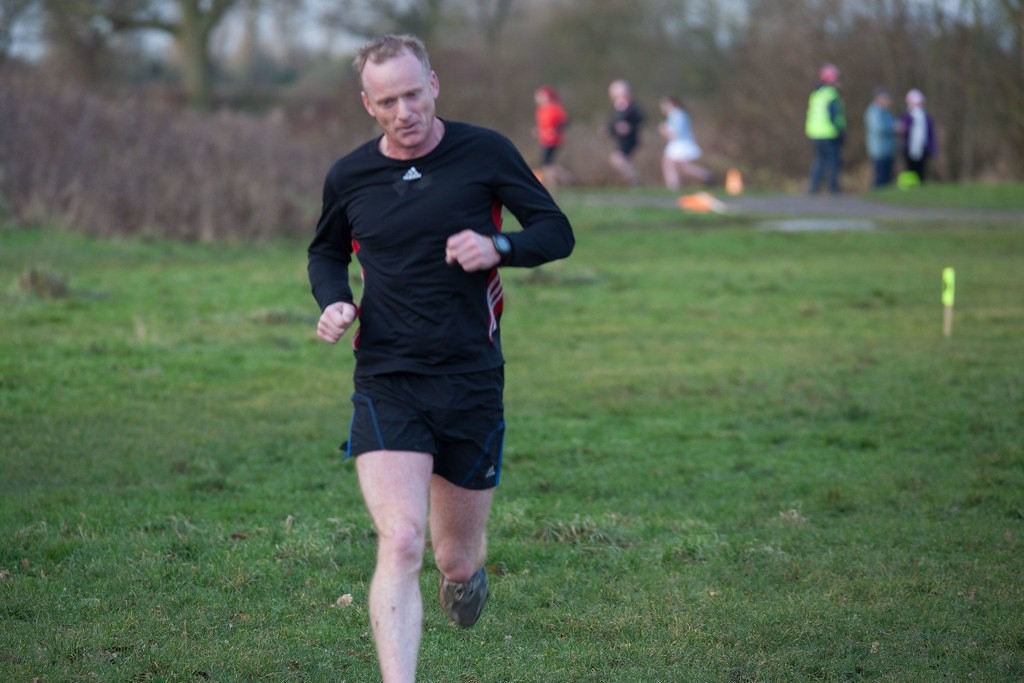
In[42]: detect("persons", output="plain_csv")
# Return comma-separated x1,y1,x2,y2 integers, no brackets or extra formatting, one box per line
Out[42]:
533,85,573,190
657,96,719,187
608,82,647,183
308,35,576,683
899,88,937,184
805,67,847,194
864,89,902,189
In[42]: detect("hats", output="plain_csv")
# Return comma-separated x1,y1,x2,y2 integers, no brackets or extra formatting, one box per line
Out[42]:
540,85,556,104
821,67,839,82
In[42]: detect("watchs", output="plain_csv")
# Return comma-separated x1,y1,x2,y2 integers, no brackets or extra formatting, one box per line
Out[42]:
487,232,511,269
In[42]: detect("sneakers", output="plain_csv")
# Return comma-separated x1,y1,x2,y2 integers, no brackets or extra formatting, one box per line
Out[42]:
438,567,487,627
